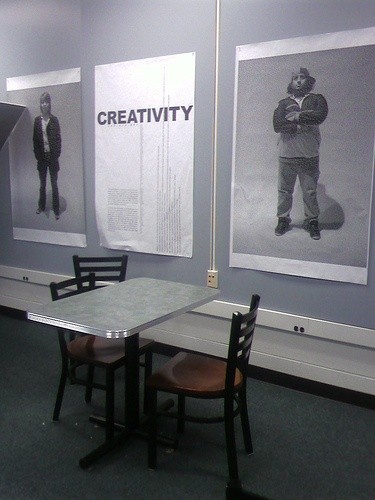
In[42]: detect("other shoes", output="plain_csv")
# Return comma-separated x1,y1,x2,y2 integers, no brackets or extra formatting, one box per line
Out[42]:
35,206,44,214
274,216,292,235
305,219,321,240
54,207,60,220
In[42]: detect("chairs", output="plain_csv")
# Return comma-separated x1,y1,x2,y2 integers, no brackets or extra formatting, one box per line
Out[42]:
49,254,152,434
146,294,260,486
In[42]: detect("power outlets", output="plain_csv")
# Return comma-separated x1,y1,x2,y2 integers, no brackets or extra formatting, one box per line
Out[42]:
207,270,219,289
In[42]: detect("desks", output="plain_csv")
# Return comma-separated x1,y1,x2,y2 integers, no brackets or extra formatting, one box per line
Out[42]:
27,277,220,471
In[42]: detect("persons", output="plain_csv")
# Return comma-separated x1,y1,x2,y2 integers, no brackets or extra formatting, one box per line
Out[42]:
271,67,328,240
33,92,62,220
